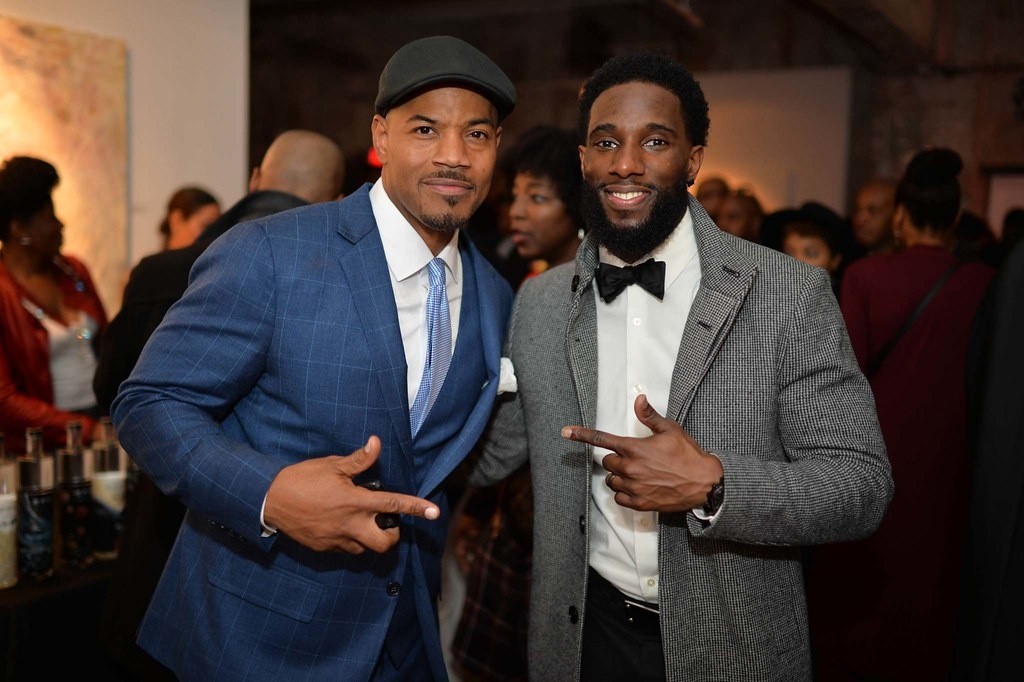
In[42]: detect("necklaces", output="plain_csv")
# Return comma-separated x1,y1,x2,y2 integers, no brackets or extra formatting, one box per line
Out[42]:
22,258,92,340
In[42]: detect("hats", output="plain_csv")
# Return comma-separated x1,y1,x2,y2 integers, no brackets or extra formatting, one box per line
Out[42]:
375,35,516,123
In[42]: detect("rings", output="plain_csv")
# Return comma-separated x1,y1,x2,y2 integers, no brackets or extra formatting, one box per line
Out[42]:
608,476,614,489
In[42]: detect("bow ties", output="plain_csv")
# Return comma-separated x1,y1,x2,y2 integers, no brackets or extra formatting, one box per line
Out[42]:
594,258,667,304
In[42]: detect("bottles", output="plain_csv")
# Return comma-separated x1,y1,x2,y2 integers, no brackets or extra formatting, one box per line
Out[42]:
0,434,21,588
92,417,128,562
54,420,94,574
16,425,52,584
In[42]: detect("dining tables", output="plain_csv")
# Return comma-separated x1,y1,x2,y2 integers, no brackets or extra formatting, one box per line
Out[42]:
0,563,109,682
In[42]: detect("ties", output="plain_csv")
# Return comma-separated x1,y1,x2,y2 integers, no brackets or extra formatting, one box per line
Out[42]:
408,258,455,442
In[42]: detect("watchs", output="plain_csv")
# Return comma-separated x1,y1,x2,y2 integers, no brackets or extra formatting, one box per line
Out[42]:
703,475,723,515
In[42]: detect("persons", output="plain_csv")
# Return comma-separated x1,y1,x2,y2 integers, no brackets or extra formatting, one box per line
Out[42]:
357,151,515,275
113,37,515,682
0,156,112,452
496,124,586,280
91,130,346,582
697,177,1022,255
791,144,991,682
459,52,896,682
850,184,897,255
781,200,847,271
158,188,219,251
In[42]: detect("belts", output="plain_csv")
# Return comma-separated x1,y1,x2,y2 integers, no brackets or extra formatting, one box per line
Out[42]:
587,573,660,632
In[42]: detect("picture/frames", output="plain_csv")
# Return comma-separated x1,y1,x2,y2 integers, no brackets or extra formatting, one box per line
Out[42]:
1,14,132,321
